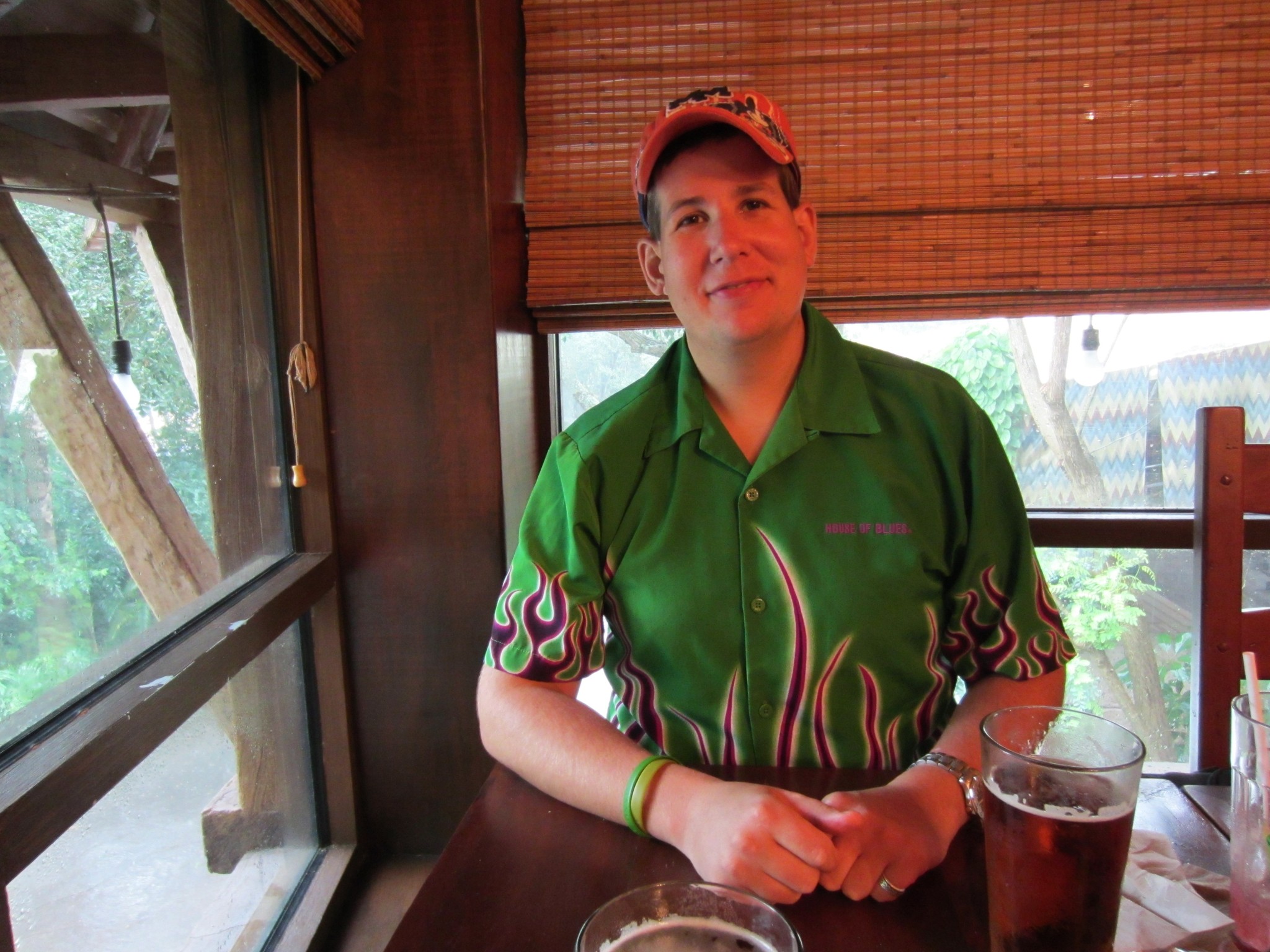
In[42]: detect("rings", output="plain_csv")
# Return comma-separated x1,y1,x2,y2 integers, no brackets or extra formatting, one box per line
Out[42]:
879,875,905,900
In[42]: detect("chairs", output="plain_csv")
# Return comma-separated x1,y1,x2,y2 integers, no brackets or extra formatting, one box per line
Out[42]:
1187,399,1270,773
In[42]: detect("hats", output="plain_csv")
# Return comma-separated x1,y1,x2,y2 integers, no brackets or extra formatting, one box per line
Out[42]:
630,86,801,231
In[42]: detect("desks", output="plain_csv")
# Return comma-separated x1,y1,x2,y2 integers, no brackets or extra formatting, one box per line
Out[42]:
364,736,1270,952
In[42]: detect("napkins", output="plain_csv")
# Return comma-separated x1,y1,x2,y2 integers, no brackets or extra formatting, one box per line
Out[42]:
1112,828,1238,952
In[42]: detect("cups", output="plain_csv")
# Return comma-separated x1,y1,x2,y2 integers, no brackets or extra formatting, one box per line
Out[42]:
1229,692,1270,952
574,880,806,952
978,704,1147,952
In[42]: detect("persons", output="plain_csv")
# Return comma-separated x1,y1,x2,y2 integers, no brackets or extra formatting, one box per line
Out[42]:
474,86,1075,909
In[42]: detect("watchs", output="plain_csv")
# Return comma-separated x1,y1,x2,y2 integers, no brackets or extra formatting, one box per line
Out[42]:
904,751,986,823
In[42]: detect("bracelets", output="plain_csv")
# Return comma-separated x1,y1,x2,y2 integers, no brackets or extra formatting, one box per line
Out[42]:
625,754,677,838
